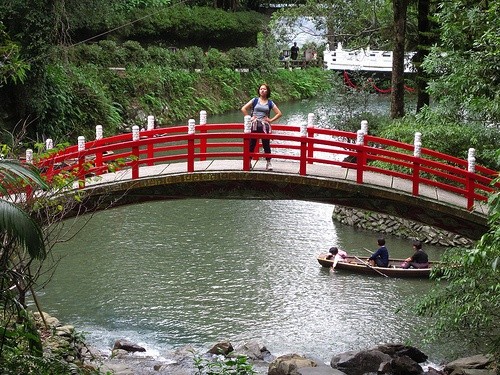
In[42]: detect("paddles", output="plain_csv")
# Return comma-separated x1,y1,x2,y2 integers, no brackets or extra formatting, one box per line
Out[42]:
364,248,380,257
354,255,389,278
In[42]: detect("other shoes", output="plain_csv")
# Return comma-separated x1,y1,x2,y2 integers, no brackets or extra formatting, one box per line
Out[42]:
266,163,273,169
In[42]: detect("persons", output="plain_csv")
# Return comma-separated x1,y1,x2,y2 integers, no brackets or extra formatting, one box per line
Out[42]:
290,43,300,70
279,52,286,64
302,50,317,67
367,239,388,268
329,247,346,271
394,242,428,269
241,84,282,170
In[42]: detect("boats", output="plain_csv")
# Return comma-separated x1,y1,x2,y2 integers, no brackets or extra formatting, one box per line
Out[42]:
317,252,463,280
